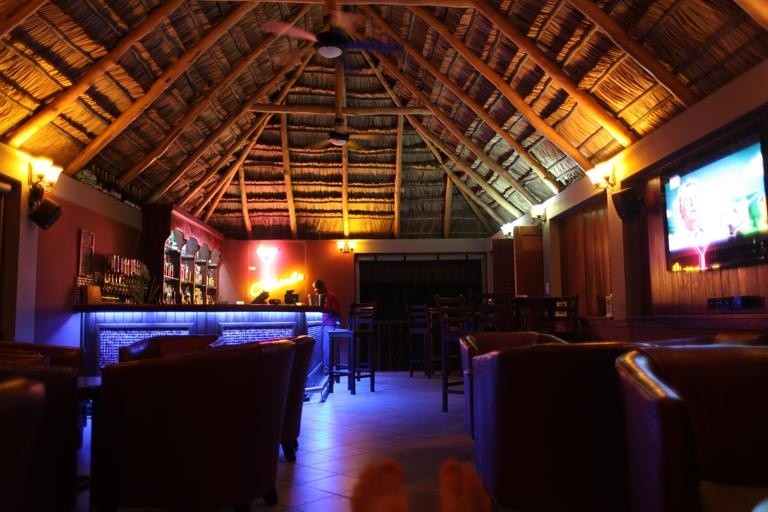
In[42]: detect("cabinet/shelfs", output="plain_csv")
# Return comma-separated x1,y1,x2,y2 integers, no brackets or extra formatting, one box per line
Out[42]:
146,203,225,305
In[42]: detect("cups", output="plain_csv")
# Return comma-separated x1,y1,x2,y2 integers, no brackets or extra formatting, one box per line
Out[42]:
318,294,326,307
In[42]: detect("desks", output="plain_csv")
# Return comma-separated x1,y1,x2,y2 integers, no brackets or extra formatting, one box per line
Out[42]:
71,376,103,407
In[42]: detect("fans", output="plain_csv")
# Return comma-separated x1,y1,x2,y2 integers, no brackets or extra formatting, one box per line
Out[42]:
262,0,405,66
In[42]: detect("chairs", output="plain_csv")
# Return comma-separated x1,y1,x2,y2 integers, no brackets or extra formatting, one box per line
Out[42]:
119,335,226,363
407,304,432,377
474,294,579,338
433,293,473,413
614,342,767,511
1,342,83,511
467,341,659,512
328,302,378,395
459,331,569,441
92,341,297,512
279,335,314,460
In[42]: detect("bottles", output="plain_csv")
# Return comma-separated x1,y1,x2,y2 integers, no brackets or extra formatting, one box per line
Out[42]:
103,254,144,303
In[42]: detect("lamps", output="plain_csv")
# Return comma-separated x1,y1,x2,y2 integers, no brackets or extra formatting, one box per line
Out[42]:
27,157,64,208
337,240,355,253
528,203,547,226
499,223,516,238
316,30,346,58
328,118,349,146
585,161,615,188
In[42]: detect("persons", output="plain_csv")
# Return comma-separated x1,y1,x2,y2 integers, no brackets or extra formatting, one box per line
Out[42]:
669,181,730,255
308,279,342,314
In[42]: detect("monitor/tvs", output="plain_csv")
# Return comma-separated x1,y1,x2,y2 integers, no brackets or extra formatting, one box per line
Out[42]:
659,132,767,272
